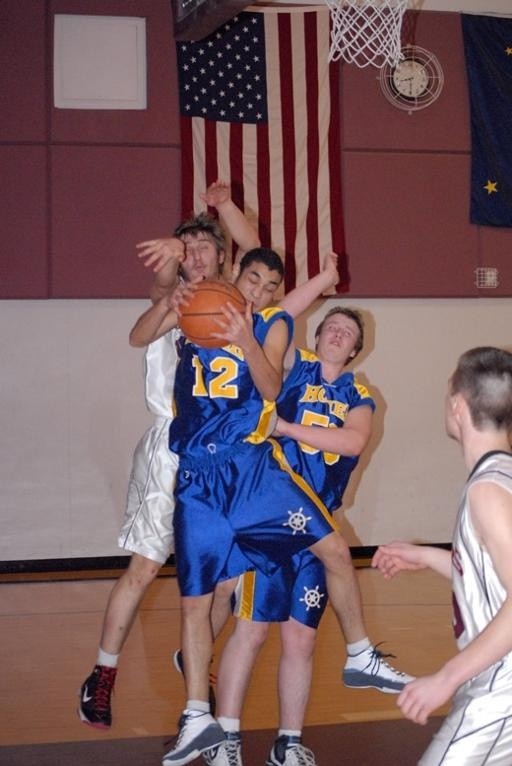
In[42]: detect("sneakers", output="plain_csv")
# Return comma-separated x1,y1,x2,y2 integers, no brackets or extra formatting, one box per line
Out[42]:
341,641,417,695
265,733,316,766
161,649,244,766
77,664,118,729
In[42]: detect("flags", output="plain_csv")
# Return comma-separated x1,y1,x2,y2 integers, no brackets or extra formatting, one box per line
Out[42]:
459,11,511,228
173,0,347,297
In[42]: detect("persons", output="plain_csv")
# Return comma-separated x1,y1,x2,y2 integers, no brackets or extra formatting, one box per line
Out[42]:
201,252,376,765
75,180,261,729
372,346,512,766
129,248,418,766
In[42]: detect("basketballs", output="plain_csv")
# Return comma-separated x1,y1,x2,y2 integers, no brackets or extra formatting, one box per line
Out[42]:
178,283,245,346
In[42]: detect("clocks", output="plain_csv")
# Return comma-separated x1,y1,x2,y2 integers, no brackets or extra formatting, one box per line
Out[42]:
389,57,432,101
